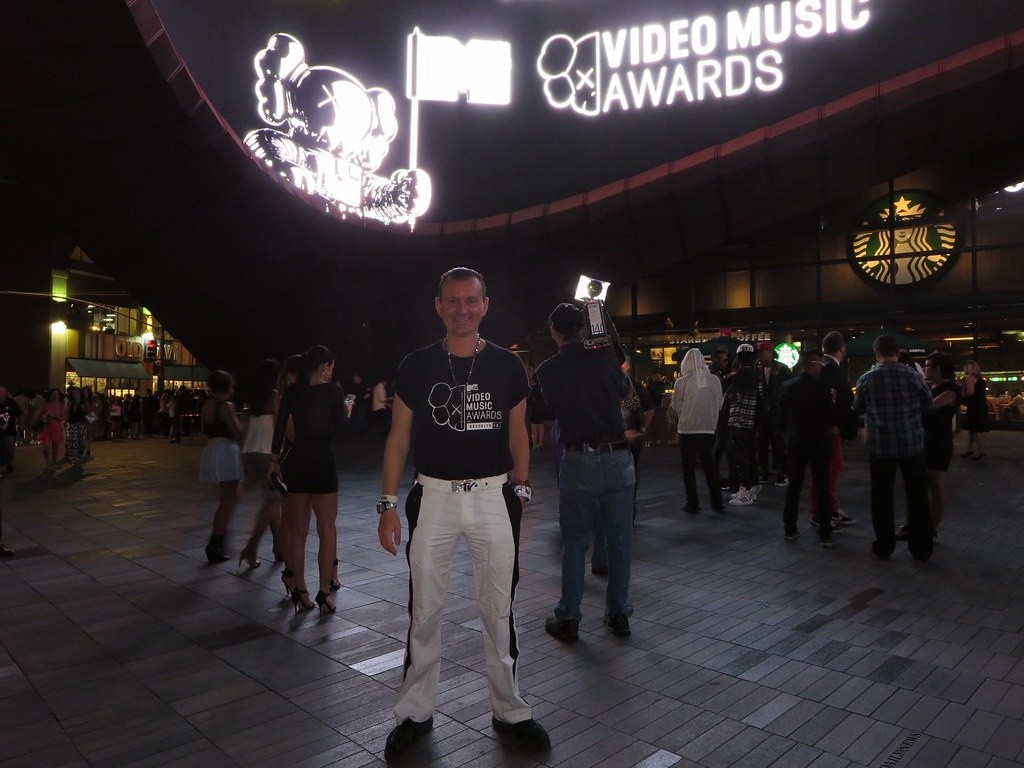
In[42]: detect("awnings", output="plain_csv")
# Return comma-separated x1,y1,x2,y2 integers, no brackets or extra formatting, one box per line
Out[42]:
68,357,151,380
158,364,212,381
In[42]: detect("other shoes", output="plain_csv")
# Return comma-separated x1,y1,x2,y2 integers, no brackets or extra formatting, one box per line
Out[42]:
601,613,633,636
782,530,809,540
590,565,610,579
820,537,841,549
384,714,436,760
543,613,582,639
0,543,15,558
204,544,233,564
0,450,95,480
490,715,551,751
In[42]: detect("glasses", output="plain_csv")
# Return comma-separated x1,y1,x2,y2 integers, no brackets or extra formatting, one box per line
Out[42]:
811,360,825,367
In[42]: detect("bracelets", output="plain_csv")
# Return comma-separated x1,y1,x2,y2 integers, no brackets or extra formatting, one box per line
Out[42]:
378,494,399,503
512,479,534,502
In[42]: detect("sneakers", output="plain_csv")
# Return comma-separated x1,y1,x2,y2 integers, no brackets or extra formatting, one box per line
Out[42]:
808,507,856,532
681,462,791,514
870,547,886,560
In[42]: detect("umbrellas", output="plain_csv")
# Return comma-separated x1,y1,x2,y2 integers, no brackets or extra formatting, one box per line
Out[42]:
670,335,749,360
846,328,930,354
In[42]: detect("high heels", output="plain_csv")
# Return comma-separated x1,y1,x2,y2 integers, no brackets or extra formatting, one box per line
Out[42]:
238,547,261,570
280,569,295,595
895,525,911,542
960,450,989,462
292,588,317,614
314,589,342,615
932,530,939,544
272,543,292,564
330,559,342,589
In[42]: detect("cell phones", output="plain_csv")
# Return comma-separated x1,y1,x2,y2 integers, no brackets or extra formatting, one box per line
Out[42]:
344,395,356,405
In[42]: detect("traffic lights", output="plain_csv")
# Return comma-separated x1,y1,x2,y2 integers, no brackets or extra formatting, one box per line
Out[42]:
147,340,157,358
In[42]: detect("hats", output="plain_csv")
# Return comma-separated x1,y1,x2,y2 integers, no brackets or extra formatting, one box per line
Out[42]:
736,344,755,355
550,303,583,333
757,341,779,360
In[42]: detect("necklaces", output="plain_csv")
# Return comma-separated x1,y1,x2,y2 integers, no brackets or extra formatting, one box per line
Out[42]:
443,332,480,430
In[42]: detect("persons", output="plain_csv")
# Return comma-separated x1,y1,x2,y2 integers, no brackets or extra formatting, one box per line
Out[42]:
90,374,181,443
376,269,552,765
523,364,548,451
590,342,654,572
961,358,990,463
0,385,96,476
334,372,394,436
532,300,639,638
239,343,356,616
199,371,247,561
667,327,961,561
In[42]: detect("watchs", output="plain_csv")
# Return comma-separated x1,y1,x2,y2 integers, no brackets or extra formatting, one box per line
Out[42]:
377,502,397,514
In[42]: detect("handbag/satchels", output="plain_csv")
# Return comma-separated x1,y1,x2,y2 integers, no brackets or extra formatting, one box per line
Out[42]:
83,411,98,425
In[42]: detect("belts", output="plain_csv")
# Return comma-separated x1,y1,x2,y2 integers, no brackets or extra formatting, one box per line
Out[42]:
414,472,511,494
570,441,636,454
